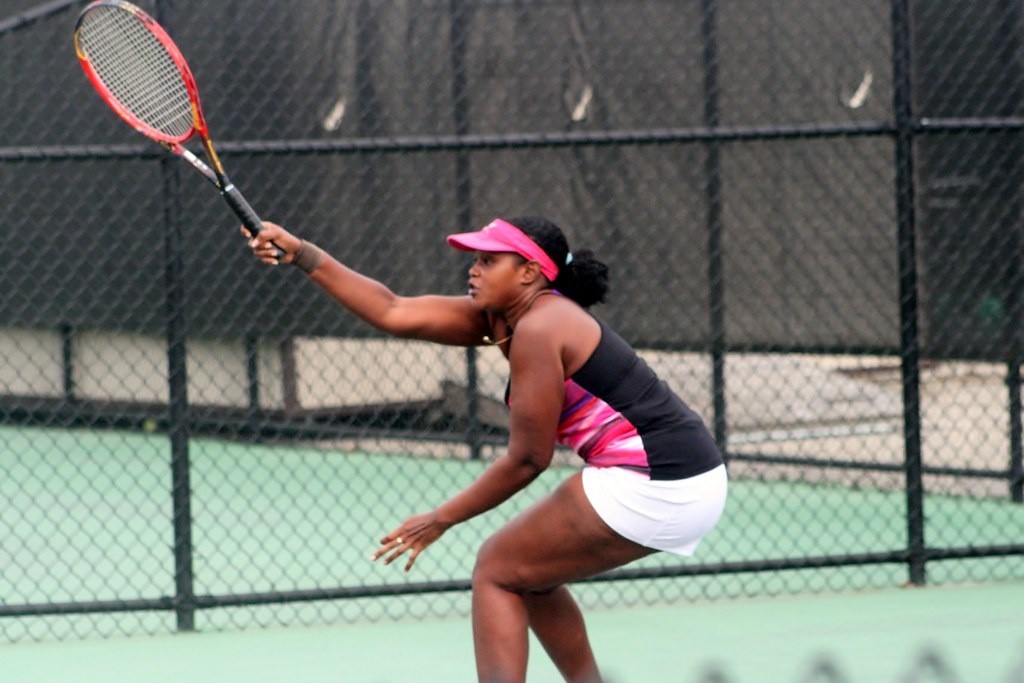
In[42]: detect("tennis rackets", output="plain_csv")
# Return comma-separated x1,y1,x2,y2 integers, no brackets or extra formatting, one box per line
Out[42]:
71,0,287,261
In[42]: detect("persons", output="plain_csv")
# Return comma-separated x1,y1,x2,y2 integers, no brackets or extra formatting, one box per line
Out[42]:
240,211,729,682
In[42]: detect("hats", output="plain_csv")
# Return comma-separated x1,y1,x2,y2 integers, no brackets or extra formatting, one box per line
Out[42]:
446,218,559,282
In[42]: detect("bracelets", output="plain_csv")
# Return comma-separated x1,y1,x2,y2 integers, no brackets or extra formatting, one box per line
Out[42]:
293,238,324,275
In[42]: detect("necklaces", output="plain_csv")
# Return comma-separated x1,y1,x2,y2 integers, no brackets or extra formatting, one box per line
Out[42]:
483,321,514,344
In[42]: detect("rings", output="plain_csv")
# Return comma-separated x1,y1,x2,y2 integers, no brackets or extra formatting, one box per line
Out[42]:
397,536,404,545
